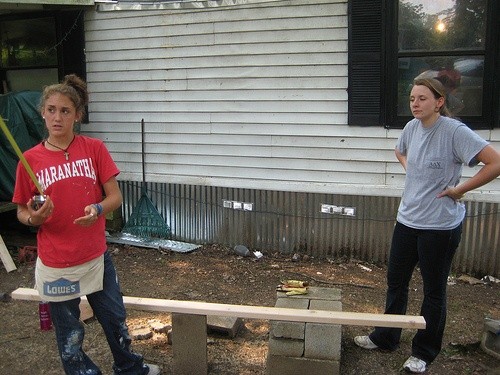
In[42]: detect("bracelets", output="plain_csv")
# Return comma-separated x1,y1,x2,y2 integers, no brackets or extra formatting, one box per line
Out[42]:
28,217,32,224
95,204,102,216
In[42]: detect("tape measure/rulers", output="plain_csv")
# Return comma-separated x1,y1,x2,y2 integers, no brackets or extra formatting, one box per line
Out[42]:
0,118,47,210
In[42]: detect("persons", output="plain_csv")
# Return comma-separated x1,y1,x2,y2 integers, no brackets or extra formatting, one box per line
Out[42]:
353,79,500,375
13,75,160,375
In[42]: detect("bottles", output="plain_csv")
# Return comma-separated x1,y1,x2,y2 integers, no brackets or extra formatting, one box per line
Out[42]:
39,302,52,331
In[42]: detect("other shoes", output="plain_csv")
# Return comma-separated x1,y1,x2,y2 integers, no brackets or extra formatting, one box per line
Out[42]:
148,364,160,375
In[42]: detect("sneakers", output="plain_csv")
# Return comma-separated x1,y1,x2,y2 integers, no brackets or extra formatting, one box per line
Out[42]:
403,356,427,373
354,335,377,348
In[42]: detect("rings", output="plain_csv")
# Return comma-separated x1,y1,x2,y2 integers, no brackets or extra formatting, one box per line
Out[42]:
91,214,94,216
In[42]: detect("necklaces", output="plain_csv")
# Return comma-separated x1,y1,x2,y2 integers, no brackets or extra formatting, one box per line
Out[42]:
47,136,75,160
